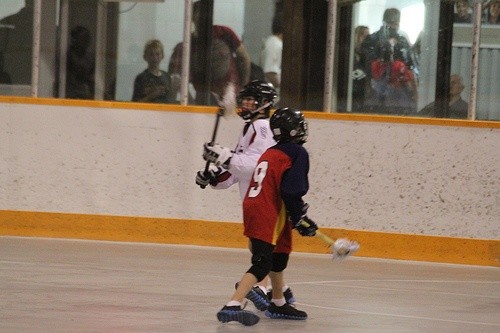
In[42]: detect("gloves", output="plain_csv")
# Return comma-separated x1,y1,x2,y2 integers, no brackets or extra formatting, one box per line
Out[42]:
203,142,231,167
196,169,216,189
297,215,318,236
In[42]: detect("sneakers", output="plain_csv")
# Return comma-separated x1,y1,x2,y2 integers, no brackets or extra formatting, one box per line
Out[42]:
216,305,260,327
235,281,268,310
265,302,307,319
267,287,295,305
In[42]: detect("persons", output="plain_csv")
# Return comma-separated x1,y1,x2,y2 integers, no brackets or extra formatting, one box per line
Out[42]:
217,107,318,326
116,0,500,119
195,78,295,312
66,25,96,101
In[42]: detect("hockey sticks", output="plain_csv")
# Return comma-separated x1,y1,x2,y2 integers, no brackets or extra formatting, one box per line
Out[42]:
200,82,237,189
301,221,360,260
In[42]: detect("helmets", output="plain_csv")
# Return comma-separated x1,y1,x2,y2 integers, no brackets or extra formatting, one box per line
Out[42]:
236,79,276,120
270,107,308,144
